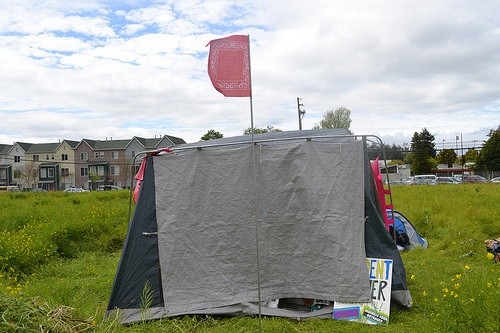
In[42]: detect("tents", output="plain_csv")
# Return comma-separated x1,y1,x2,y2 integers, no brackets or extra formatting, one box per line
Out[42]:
385,211,430,252
105,128,414,325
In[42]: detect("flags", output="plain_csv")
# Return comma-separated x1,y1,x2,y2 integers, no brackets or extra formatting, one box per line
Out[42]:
205,35,250,97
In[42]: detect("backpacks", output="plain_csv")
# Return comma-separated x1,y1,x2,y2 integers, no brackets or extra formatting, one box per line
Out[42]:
485,238,500,264
389,225,410,252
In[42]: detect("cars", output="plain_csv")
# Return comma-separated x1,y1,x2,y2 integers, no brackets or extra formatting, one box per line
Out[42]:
466,175,500,183
63,187,89,192
96,185,123,190
436,174,463,184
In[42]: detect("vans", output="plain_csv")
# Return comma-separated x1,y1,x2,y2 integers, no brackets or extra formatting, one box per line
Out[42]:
414,174,437,184
